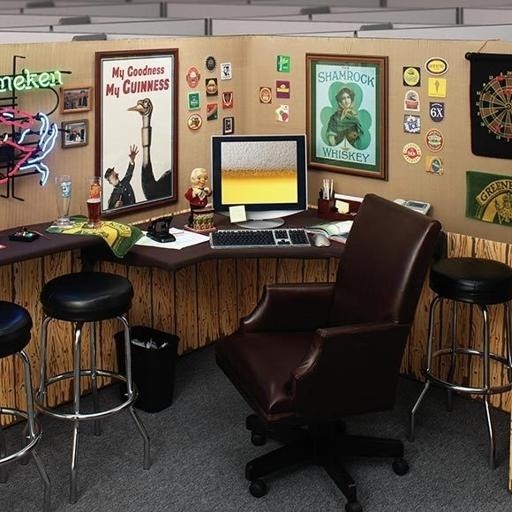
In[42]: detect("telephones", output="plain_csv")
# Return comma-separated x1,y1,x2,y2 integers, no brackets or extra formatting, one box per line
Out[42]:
394,198,431,215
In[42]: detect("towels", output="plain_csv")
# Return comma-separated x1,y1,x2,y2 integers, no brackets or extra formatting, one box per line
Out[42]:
46,216,144,260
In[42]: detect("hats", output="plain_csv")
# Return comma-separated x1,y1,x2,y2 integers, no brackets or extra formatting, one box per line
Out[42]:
104,167,115,180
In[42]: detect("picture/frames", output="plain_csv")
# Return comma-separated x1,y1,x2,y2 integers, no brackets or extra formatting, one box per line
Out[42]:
60,85,94,116
221,117,235,136
92,46,181,220
61,118,90,149
304,50,392,182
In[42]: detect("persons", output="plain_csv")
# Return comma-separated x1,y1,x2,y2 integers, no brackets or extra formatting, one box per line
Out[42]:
104,144,141,210
326,88,364,146
185,166,212,227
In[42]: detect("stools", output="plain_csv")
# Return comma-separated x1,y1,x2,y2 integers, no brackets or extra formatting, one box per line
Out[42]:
406,251,512,469
1,301,55,510
23,270,155,503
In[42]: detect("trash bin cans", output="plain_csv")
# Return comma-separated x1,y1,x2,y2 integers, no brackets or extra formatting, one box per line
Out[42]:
113,325,180,413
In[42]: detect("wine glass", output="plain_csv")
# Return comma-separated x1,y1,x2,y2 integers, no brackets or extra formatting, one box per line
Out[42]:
54,175,72,226
83,177,103,229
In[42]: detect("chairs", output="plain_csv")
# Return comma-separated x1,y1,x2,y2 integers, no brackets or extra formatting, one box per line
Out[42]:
210,189,449,511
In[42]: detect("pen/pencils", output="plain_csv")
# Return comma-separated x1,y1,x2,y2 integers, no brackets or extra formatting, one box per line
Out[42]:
319,178,334,200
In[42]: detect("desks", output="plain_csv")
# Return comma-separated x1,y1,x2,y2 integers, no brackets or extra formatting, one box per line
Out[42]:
1,217,120,267
109,205,447,272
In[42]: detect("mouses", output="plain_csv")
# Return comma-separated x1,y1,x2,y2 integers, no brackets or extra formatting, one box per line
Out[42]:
312,235,329,246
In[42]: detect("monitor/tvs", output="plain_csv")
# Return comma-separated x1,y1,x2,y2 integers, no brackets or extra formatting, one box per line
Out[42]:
210,134,308,229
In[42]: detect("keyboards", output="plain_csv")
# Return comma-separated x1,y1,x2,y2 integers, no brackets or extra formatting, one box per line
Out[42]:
209,227,310,249
